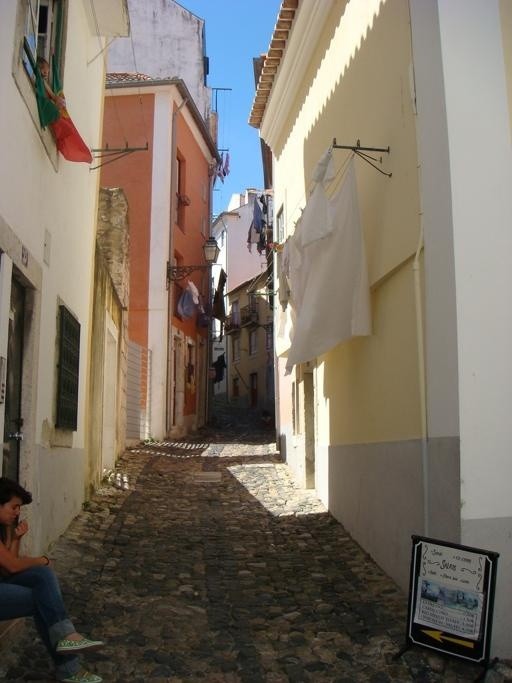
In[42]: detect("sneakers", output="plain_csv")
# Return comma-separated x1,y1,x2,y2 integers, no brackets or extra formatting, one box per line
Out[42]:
61,670,103,683
56,638,105,656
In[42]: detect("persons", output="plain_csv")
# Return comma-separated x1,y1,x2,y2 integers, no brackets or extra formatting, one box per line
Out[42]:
0,476,106,683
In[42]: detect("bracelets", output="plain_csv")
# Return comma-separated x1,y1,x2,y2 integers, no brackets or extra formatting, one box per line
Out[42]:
42,555,49,565
